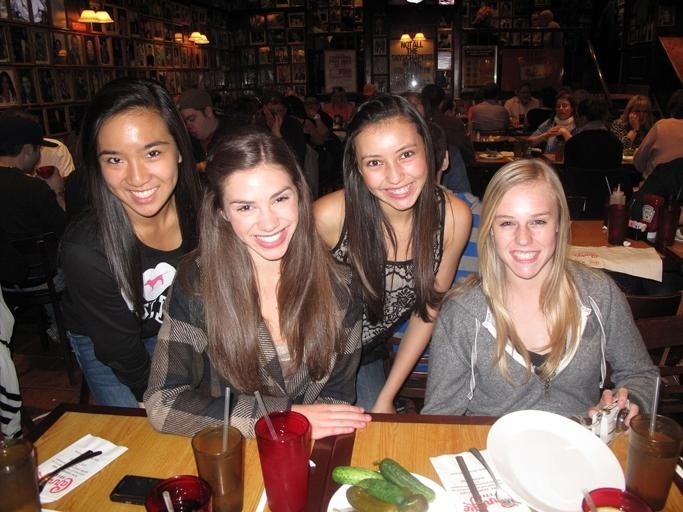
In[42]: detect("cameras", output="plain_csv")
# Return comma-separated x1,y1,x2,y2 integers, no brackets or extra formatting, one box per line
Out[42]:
592,399,630,445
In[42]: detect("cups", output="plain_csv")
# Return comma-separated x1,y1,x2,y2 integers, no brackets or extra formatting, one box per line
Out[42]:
553,141,564,160
142,474,213,512
622,412,683,510
36,165,54,180
0,439,41,512
581,487,652,511
253,411,311,510
191,426,246,512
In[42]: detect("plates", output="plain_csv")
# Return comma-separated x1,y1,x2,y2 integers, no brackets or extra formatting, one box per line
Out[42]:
479,154,504,160
325,473,448,512
484,407,626,511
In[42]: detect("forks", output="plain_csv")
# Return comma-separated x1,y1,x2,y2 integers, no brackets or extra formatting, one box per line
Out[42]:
470,445,521,512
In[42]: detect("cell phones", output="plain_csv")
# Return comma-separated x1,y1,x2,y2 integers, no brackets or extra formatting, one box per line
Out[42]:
110,474,167,506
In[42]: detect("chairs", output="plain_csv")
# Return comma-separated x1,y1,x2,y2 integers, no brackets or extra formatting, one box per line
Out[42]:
382,332,435,414
0,228,83,390
527,106,555,129
626,295,683,415
559,129,624,217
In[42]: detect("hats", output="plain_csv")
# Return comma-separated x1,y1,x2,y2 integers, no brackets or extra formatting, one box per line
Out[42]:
0,111,58,150
176,88,213,110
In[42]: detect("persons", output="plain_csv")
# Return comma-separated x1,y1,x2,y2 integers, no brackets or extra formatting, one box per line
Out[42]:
53,80,205,408
141,124,374,443
307,91,474,415
463,6,565,49
419,156,664,434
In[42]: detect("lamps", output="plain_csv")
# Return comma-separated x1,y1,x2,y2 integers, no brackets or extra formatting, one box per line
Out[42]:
76,0,118,25
186,28,210,45
398,25,427,42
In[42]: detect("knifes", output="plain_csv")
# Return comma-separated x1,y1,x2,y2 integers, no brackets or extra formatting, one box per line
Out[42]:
453,454,490,512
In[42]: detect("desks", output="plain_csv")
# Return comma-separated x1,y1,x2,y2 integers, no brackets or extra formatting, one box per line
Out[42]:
471,151,636,183
446,219,681,281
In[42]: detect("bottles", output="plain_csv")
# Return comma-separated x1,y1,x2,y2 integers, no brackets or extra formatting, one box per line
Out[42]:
641,194,680,245
608,183,627,244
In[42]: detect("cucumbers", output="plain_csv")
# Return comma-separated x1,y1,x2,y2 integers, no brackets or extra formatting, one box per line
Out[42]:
332,459,435,512
